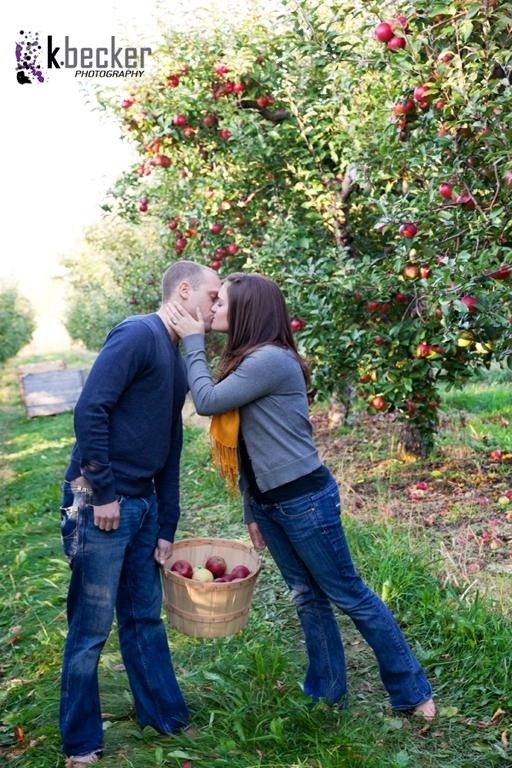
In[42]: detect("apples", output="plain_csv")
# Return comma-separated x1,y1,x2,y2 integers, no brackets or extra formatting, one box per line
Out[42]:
478,497,491,506
121,0,510,409
411,491,424,499
417,480,430,492
170,555,251,582
499,496,511,505
491,449,503,461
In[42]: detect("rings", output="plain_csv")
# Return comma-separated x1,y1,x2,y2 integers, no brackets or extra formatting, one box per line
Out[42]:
173,319,178,324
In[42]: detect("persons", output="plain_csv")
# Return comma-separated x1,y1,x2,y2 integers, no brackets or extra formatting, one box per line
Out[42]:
57,259,224,766
162,271,436,723
